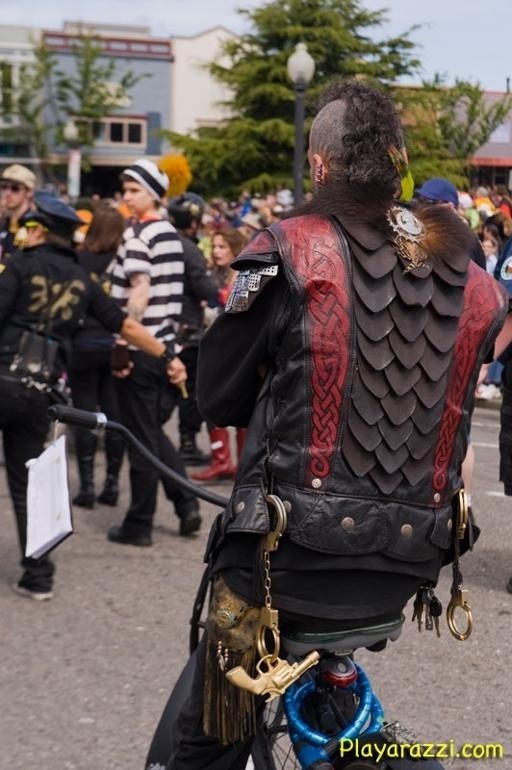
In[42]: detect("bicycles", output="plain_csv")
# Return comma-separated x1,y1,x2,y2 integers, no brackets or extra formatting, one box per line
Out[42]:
33,395,419,769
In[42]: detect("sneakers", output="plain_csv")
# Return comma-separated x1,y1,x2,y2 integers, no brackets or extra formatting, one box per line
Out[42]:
8,575,53,602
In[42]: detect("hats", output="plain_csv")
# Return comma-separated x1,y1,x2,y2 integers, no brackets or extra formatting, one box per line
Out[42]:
1,163,36,189
121,158,170,205
29,192,87,238
413,179,460,208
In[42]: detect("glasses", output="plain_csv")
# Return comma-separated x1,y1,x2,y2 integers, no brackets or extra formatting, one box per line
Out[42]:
1,182,21,194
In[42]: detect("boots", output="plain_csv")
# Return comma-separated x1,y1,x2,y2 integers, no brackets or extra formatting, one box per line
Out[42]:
192,426,249,483
175,432,214,467
97,438,121,508
74,444,96,509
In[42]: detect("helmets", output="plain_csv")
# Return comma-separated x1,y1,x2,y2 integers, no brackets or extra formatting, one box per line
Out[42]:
168,192,208,229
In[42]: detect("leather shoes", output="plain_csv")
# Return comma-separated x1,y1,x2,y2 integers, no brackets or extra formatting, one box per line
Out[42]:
107,521,157,547
175,509,204,536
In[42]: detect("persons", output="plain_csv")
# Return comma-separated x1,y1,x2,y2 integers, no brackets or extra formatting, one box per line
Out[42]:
2,84,512,770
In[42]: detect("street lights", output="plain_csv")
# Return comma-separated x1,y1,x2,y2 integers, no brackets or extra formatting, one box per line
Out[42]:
283,36,319,208
61,114,81,201
